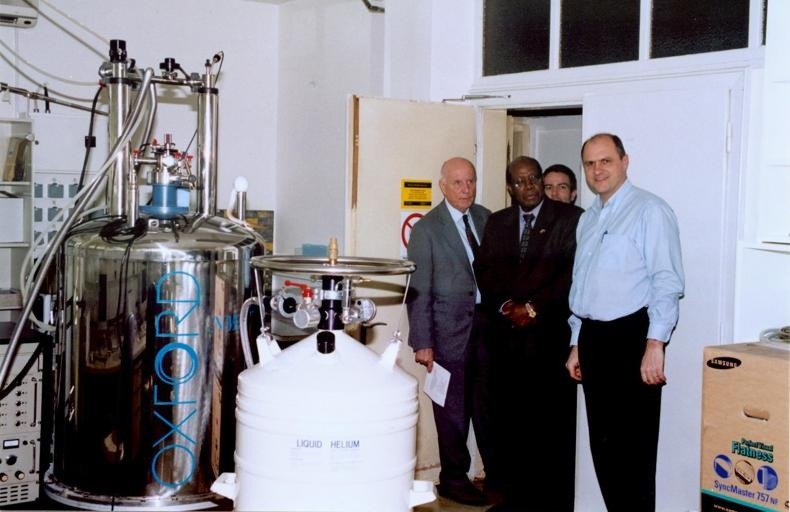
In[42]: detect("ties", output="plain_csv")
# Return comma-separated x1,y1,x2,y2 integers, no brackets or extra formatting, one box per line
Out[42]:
462,214,479,259
519,213,536,266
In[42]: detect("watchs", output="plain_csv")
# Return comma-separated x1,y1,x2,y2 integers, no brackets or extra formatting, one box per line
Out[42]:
523,302,537,320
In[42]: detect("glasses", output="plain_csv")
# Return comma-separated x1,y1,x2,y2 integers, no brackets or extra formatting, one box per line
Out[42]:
508,174,543,191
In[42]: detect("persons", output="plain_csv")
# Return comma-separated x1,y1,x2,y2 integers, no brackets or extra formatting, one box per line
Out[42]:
474,153,586,511
407,157,505,506
542,163,578,206
564,134,684,512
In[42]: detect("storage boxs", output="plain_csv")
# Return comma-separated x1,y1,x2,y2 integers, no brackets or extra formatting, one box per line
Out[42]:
700,491,776,512
700,342,790,509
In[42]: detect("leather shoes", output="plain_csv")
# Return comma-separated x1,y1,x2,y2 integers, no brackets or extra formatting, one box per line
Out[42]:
437,473,491,508
